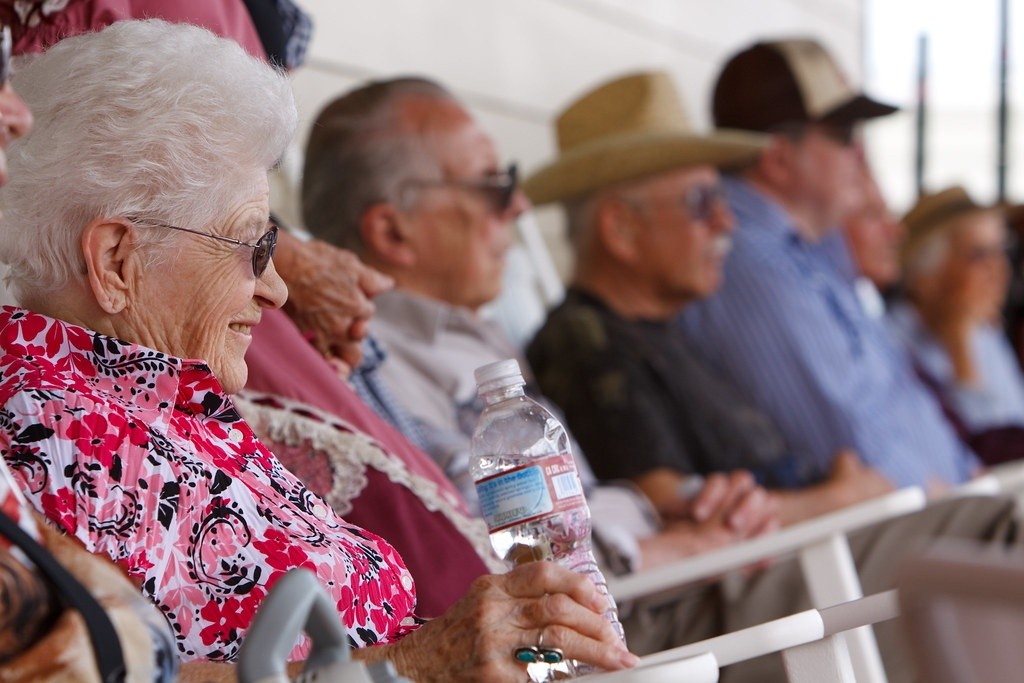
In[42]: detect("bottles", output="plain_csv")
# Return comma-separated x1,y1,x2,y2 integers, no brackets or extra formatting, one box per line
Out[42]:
468,358,628,683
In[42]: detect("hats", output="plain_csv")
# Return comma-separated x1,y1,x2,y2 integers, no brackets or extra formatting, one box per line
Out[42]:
898,185,1010,297
521,71,774,202
716,38,899,132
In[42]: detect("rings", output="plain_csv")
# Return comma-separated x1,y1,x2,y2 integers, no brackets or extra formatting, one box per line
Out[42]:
512,630,564,665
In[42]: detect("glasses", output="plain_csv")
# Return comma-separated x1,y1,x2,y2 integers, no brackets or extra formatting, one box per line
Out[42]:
84,216,279,278
614,183,724,220
389,163,519,222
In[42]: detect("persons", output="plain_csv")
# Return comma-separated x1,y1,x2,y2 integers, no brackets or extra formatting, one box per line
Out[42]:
522,35,1024,524
0,17,642,683
0,0,518,627
0,27,178,683
298,76,783,582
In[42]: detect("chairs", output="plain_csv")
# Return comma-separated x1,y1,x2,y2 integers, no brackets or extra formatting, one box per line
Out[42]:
288,224,927,683
475,207,1024,524
545,607,826,683
